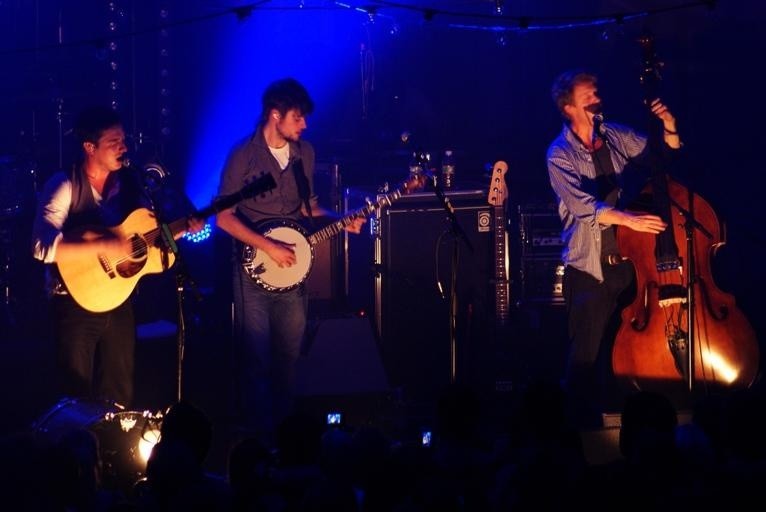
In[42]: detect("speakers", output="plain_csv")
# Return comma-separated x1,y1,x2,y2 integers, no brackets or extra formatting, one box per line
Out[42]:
306,163,341,301
520,212,568,312
375,190,513,395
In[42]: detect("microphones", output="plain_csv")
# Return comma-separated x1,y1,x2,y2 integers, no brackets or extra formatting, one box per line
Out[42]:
593,114,604,137
401,131,429,171
122,158,162,187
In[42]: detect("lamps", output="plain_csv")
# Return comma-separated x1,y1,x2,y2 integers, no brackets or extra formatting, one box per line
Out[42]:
90,402,170,473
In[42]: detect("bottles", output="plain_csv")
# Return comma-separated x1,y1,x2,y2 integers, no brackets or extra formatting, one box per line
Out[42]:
407,140,457,191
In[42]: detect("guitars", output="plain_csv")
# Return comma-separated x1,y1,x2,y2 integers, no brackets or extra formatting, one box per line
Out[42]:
462,160,550,448
54,170,277,313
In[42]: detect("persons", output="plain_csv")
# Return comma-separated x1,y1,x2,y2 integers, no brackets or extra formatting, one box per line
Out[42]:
543,69,683,392
27,100,207,417
211,76,370,411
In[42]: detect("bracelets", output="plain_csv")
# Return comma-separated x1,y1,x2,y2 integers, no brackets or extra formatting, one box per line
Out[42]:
662,126,681,137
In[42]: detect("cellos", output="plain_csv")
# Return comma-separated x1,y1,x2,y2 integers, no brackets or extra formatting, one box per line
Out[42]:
613,23,761,408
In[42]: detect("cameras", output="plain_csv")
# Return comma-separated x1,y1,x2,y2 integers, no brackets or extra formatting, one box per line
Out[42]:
326,412,343,428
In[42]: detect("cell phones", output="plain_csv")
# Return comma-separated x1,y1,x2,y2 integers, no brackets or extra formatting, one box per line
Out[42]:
418,431,433,453
